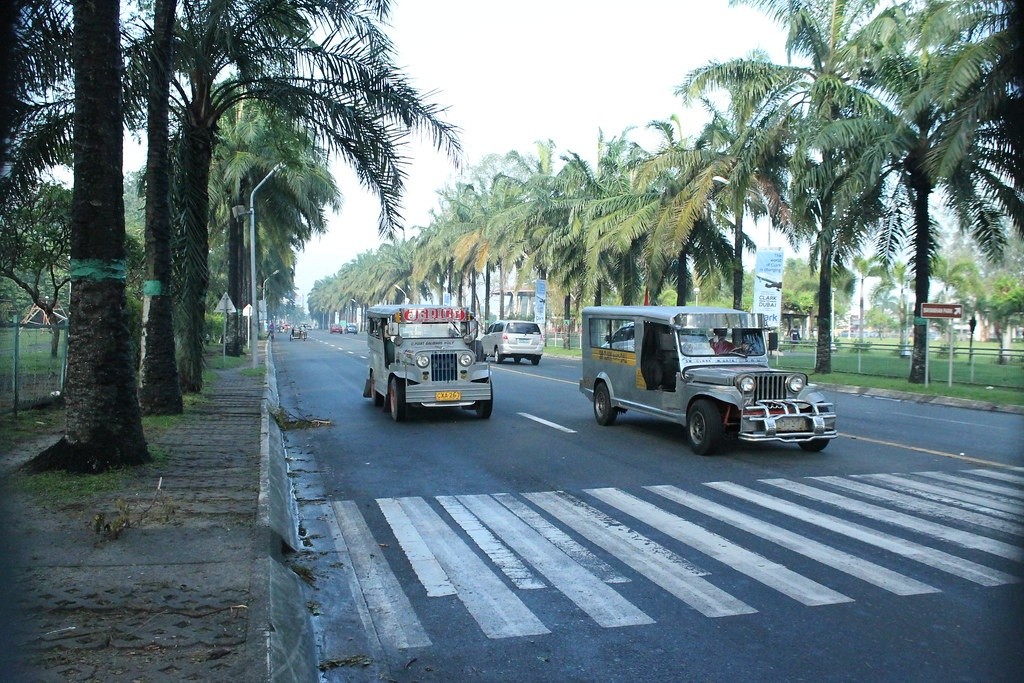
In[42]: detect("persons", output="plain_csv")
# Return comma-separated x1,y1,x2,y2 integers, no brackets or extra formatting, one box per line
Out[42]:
300,325,305,336
709,328,750,354
267,321,274,339
789,324,801,352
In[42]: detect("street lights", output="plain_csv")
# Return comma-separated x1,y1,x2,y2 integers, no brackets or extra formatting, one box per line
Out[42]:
394,283,407,305
230,161,288,370
351,299,361,333
322,307,330,329
711,174,771,323
262,270,280,339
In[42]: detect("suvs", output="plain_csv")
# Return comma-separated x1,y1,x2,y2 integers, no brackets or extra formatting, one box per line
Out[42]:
481,319,545,366
361,302,494,422
578,303,839,457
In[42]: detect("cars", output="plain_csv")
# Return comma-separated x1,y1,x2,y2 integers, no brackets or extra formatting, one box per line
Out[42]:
330,325,342,334
344,323,359,334
600,320,635,353
277,323,313,342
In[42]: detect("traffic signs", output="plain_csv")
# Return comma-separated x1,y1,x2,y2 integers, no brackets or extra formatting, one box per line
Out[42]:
920,302,963,319
